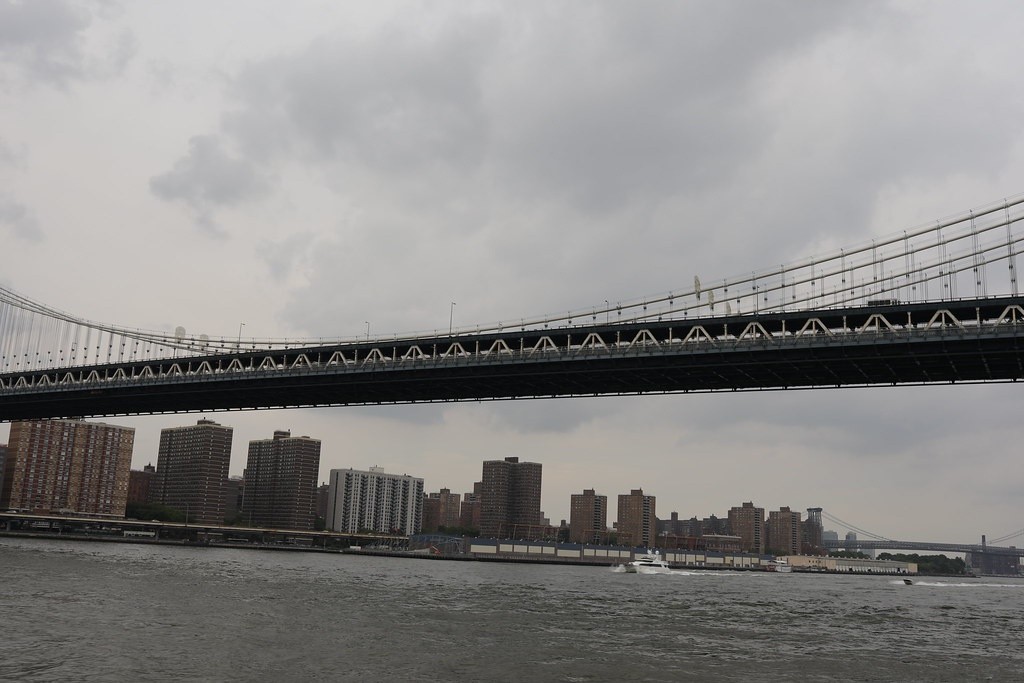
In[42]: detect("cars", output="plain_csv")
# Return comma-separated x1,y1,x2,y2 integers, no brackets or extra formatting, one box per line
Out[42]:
209,539,217,543
152,520,159,522
200,537,208,542
276,539,288,544
5,509,17,514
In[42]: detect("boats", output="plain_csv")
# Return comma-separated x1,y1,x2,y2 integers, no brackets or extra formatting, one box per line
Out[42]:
903,578,913,585
623,551,670,573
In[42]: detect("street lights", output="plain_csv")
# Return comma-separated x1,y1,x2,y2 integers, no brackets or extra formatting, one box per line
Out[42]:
450,301,457,329
757,285,760,314
365,321,369,340
238,323,245,340
605,300,609,326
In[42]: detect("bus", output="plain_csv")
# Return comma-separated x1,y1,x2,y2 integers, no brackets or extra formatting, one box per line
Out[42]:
288,538,312,546
124,531,156,538
228,538,248,544
197,533,223,542
